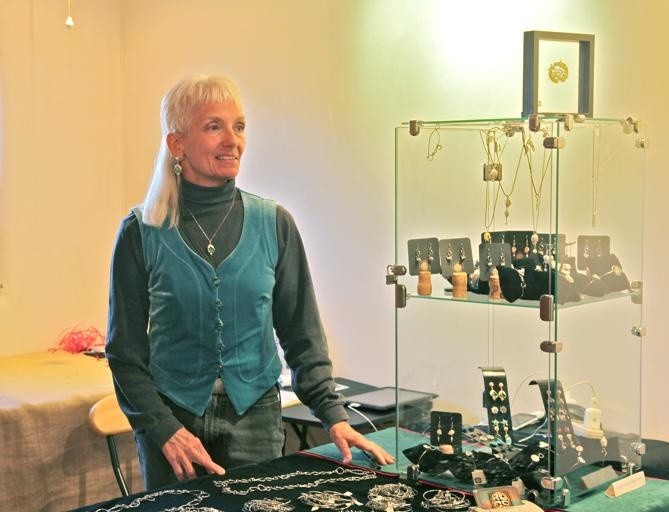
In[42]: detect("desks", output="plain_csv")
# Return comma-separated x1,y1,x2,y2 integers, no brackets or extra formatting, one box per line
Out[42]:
0,350,145,512
69,419,669,512
281,377,432,450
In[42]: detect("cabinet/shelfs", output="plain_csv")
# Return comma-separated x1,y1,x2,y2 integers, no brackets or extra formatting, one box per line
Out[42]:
396,119,647,508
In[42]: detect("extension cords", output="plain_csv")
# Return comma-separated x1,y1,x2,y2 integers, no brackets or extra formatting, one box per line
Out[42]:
535,412,603,438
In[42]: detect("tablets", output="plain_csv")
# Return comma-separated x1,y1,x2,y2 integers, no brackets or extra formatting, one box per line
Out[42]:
345,386,439,411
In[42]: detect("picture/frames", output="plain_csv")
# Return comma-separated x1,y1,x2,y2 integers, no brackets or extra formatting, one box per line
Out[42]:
521,31,595,120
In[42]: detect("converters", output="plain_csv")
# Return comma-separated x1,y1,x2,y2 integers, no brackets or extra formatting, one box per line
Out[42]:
584,408,601,429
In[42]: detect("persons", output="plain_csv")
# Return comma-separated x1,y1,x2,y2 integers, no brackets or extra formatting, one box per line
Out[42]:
104,73,397,495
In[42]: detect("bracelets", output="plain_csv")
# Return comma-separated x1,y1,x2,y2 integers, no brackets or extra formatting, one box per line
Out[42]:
583,263,626,292
198,481,471,512
512,267,527,300
426,124,443,163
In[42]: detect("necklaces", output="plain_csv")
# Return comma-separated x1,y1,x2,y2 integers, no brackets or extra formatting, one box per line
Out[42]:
475,124,555,247
592,123,603,228
179,186,238,257
209,466,379,497
95,486,210,512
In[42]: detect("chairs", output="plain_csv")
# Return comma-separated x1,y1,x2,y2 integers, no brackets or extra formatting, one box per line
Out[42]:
89,393,132,496
